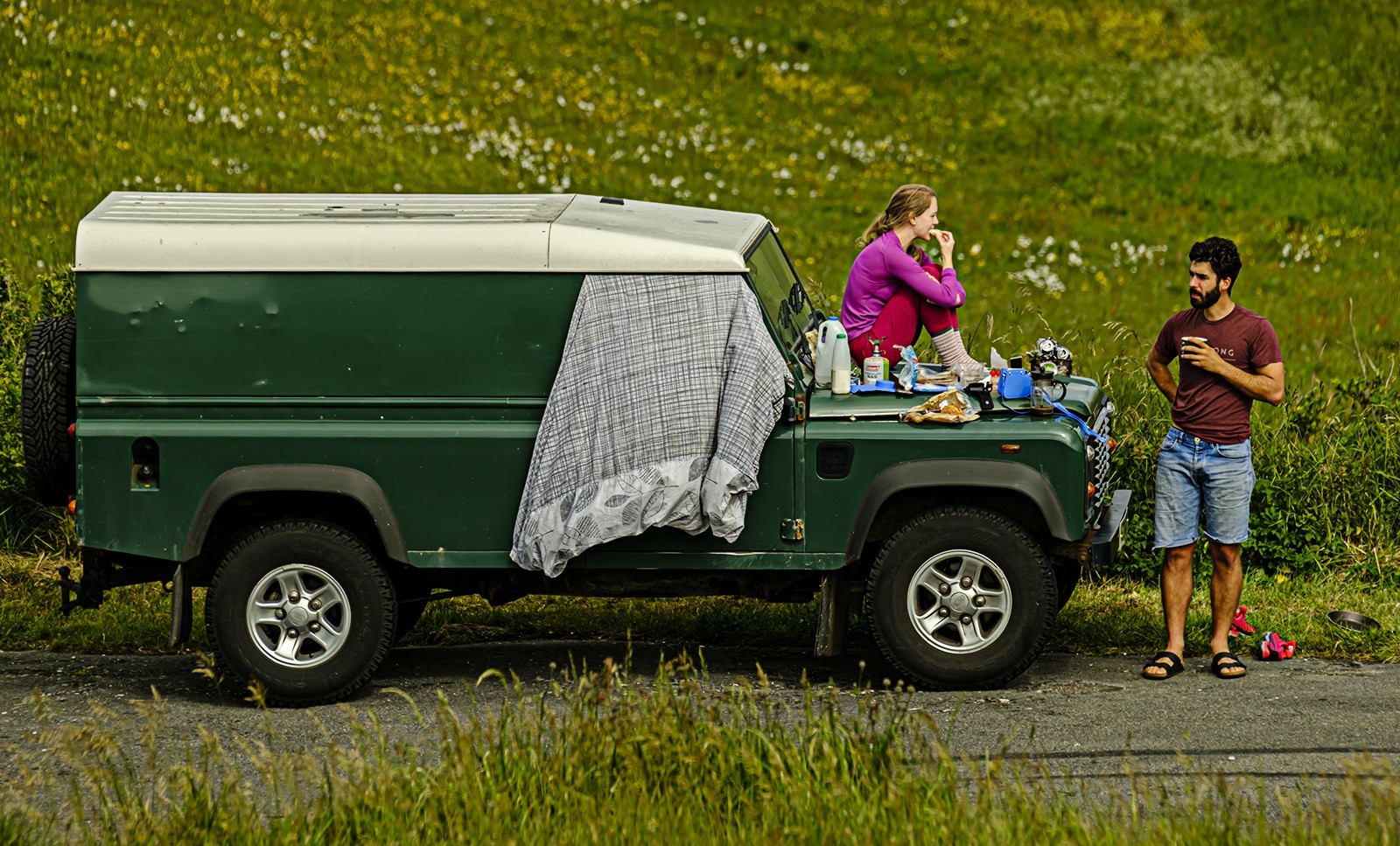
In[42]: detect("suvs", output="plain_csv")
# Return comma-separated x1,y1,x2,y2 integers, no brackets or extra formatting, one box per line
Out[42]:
50,187,1132,710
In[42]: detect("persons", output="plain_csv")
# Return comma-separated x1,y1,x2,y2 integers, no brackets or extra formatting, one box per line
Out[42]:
1142,238,1287,679
841,184,991,378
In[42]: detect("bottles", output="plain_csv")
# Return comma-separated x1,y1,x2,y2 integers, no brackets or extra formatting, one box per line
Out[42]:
831,333,850,395
815,316,847,383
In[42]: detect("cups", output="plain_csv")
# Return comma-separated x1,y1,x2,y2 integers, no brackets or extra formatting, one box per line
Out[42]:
1181,336,1208,354
1030,366,1067,417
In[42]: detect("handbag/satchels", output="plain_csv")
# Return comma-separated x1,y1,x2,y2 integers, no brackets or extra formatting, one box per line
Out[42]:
998,368,1033,399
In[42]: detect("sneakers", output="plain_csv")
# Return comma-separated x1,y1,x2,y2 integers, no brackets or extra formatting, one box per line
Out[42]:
1228,604,1258,639
1259,630,1297,661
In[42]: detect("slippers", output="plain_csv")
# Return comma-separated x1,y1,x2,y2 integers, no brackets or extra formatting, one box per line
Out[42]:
1142,651,1186,679
1210,652,1247,680
